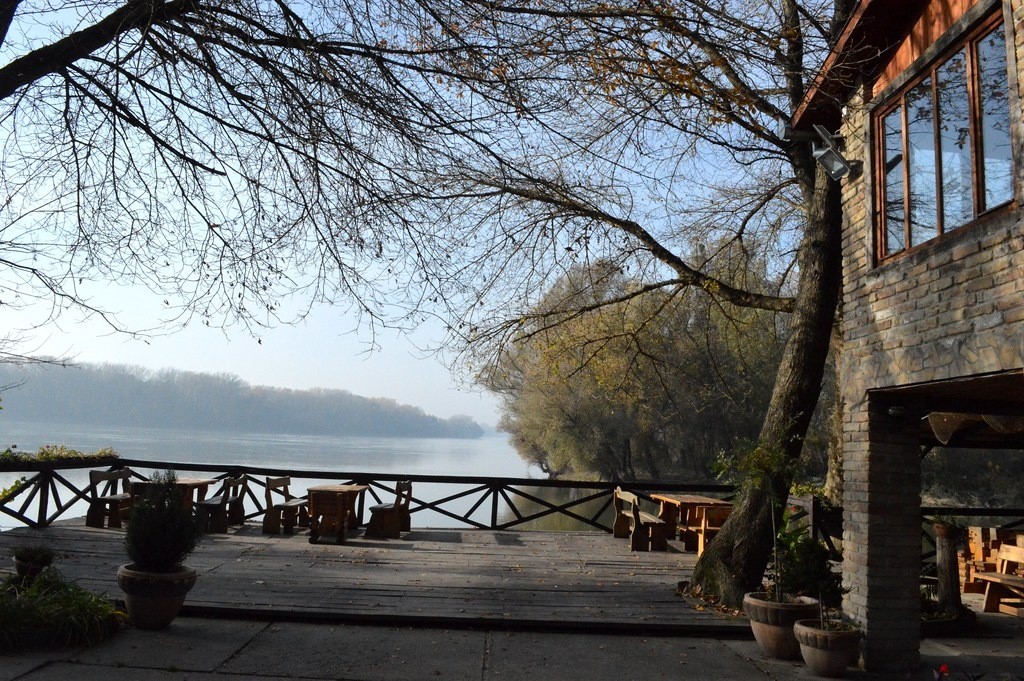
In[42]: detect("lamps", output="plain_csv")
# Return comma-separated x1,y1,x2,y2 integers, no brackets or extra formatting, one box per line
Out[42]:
812,125,851,181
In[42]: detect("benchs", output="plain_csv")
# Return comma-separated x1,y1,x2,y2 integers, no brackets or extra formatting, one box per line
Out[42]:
613,486,666,552
262,476,310,535
972,543,1024,613
364,479,412,539
197,474,249,533
85,467,134,529
687,505,735,557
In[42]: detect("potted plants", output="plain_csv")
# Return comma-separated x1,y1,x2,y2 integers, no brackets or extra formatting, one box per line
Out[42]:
117,466,198,632
793,560,860,676
930,515,957,538
743,504,820,659
12,538,53,579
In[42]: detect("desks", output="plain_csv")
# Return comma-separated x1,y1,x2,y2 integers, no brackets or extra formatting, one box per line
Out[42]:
650,494,733,551
132,477,219,525
307,484,369,544
